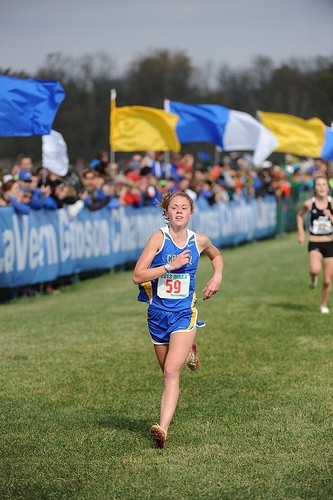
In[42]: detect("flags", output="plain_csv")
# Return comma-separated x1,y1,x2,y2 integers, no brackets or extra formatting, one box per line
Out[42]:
163,100,333,166
0,74,70,176
108,88,181,152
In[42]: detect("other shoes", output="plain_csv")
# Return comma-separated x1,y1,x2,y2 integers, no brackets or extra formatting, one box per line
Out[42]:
150,424,166,448
319,306,330,314
186,343,202,371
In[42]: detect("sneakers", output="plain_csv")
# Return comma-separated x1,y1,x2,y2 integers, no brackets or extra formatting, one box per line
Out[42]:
309,276,319,288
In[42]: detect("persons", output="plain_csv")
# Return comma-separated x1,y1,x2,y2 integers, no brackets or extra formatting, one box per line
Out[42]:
297,175,333,313
0,150,307,214
132,192,224,448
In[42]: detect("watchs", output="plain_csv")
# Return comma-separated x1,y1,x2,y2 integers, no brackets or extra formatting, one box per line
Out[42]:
164,263,172,273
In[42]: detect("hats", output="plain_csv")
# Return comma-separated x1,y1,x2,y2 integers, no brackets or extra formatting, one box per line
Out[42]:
19,168,32,182
